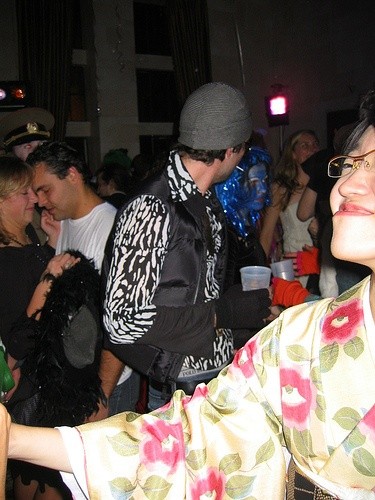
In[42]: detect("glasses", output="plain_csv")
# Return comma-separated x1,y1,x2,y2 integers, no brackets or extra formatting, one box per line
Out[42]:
327,148,375,177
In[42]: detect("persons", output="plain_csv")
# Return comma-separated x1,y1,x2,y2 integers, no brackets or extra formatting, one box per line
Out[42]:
0,122,371,500
8,91,375,500
103,82,275,415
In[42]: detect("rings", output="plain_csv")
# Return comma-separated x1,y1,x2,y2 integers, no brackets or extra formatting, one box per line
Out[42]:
66,260,72,265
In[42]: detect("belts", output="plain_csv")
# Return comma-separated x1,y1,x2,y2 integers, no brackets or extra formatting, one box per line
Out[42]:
148,378,213,396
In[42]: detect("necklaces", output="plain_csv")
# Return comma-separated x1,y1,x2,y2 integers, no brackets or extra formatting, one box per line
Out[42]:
10,236,49,266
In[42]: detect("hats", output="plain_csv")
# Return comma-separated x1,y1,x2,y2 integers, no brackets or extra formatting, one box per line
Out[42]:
177,82,253,151
0,107,55,149
282,122,320,148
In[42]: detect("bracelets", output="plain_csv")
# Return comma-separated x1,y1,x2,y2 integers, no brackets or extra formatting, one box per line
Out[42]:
40,273,55,281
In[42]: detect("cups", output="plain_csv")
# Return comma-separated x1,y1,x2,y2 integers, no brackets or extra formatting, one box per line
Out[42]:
269,260,295,281
240,266,271,290
